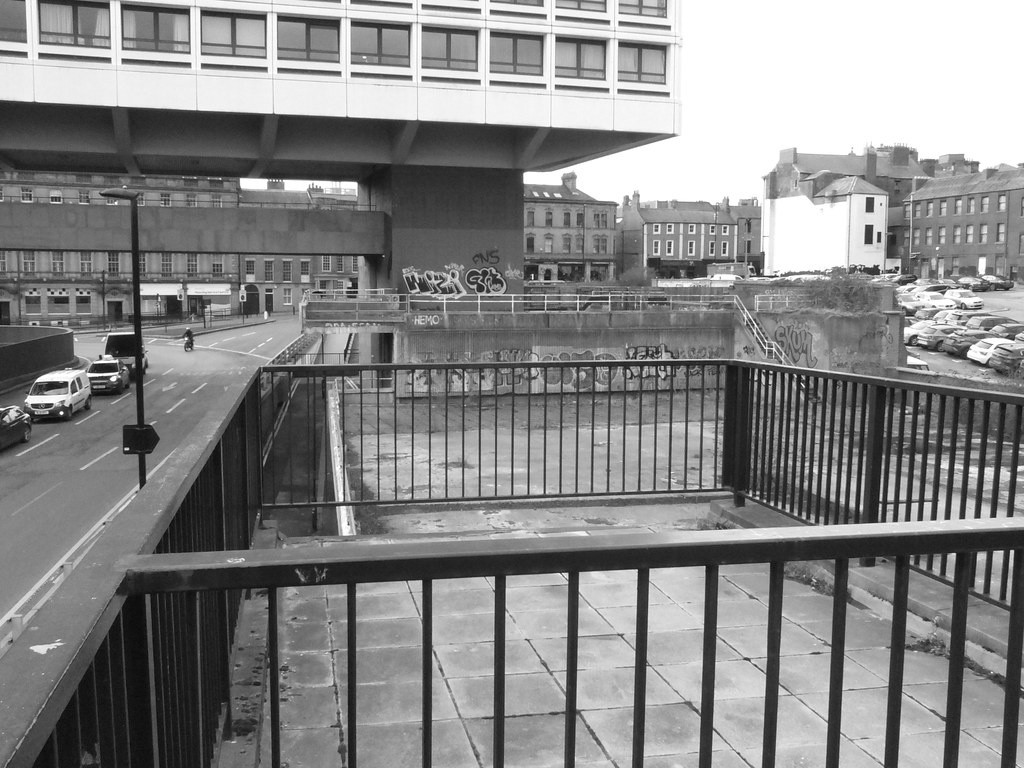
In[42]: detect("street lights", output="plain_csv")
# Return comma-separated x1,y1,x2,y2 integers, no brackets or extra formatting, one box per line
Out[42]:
99,187,146,490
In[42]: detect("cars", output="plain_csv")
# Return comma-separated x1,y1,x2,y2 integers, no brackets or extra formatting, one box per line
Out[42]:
894,294,925,316
942,310,996,327
904,319,937,347
1014,330,1024,343
914,308,941,320
85,359,131,395
941,331,1003,360
982,274,1014,291
944,289,984,310
988,323,1024,341
933,310,962,323
966,316,1021,332
0,405,32,451
872,274,951,295
916,292,957,310
956,276,991,292
938,279,965,289
917,324,967,352
990,342,1024,377
967,338,1017,369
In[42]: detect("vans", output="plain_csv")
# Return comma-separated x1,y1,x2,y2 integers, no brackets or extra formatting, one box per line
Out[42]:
23,368,92,423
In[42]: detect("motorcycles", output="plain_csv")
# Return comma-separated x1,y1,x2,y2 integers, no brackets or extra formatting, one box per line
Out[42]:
183,337,195,352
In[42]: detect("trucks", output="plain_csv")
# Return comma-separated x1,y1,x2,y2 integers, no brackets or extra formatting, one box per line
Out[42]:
706,263,757,278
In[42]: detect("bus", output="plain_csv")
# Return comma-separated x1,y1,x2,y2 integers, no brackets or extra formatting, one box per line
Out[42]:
101,332,149,375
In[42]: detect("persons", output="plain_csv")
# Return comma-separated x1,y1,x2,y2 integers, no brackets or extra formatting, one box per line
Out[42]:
182,327,193,345
